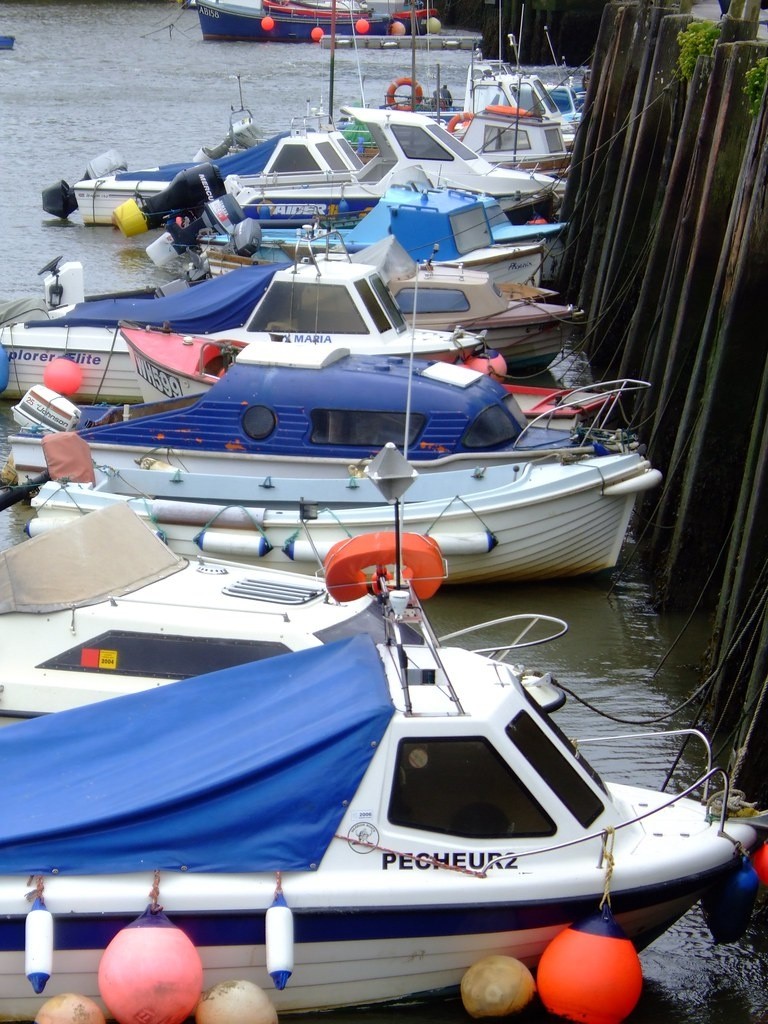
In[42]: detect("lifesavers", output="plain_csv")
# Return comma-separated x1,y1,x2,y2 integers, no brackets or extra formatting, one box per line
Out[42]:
445,111,477,135
319,527,450,605
484,103,535,119
385,75,425,113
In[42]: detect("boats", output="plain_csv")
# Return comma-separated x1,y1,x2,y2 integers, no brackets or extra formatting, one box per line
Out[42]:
0,1,668,590
0,443,758,1022
0,500,566,758
